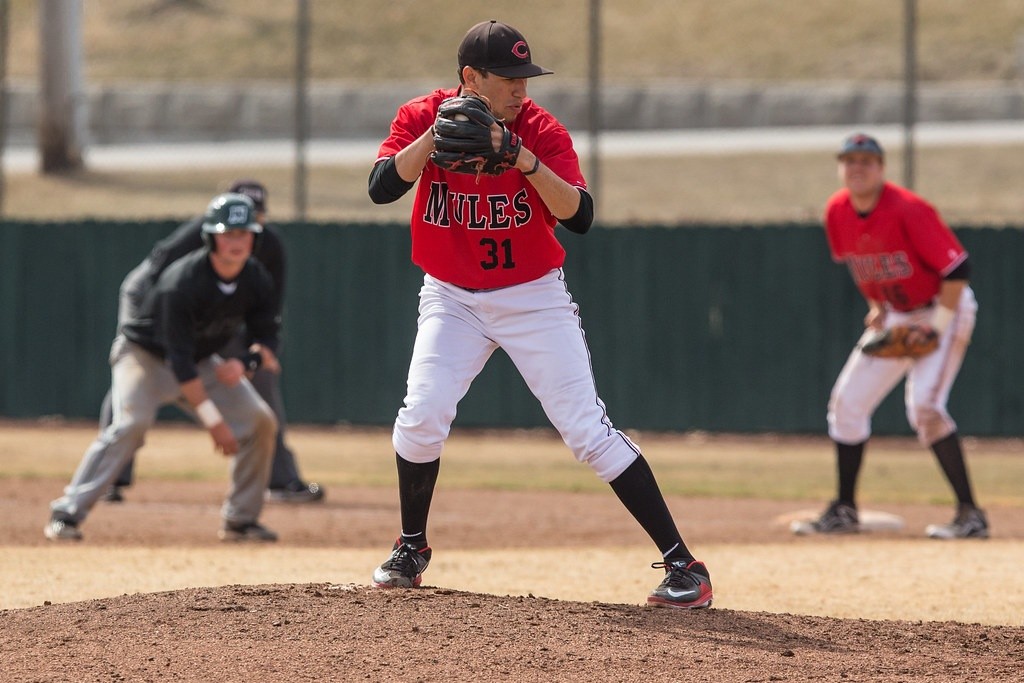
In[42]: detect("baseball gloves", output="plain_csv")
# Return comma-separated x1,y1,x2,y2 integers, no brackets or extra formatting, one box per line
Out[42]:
861,312,941,363
431,86,524,186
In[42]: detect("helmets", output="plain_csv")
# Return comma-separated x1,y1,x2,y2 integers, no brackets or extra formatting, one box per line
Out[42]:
201,193,262,253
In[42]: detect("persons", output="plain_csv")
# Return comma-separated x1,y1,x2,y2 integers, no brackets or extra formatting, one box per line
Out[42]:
788,132,995,539
42,180,326,543
364,21,717,611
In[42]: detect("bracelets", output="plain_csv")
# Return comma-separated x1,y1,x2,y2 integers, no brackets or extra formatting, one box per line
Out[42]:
430,125,435,137
194,399,223,429
928,302,953,335
522,157,539,175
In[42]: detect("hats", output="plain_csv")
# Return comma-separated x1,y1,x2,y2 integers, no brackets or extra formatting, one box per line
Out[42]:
838,134,883,159
457,20,554,78
230,182,266,213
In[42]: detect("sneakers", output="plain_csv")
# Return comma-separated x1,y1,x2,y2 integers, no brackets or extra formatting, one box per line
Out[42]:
647,559,713,609
45,522,84,542
791,500,861,535
219,522,278,542
101,485,123,501
265,480,324,503
926,503,989,539
373,539,432,587
